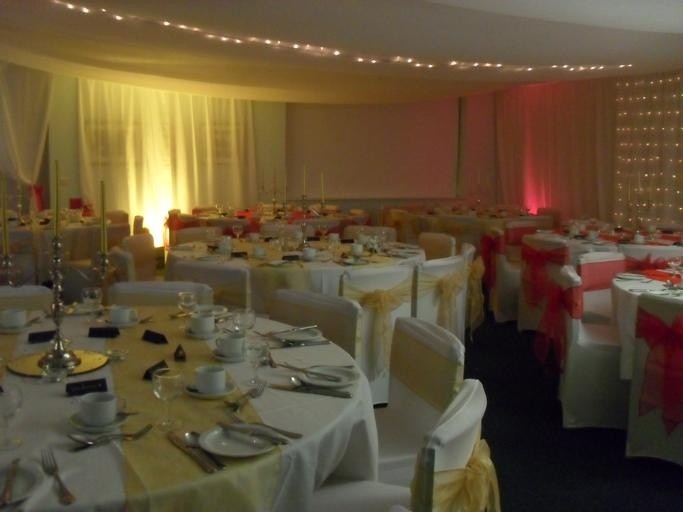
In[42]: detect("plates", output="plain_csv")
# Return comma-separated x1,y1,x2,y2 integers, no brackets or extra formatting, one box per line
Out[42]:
1,287,364,509
7,208,99,227
167,201,421,267
614,255,682,296
537,220,681,246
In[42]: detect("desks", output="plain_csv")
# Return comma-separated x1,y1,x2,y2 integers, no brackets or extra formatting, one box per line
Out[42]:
610,268,682,380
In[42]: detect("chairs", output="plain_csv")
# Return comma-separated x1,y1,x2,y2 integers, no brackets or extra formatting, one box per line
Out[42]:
415,209,682,336
558,266,631,429
381,202,556,247
625,292,682,464
1,203,501,512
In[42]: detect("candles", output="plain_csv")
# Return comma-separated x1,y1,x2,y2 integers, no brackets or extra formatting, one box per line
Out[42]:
0,178,11,256
626,171,654,201
299,163,306,195
51,155,61,238
280,169,286,203
96,178,110,252
317,171,323,201
